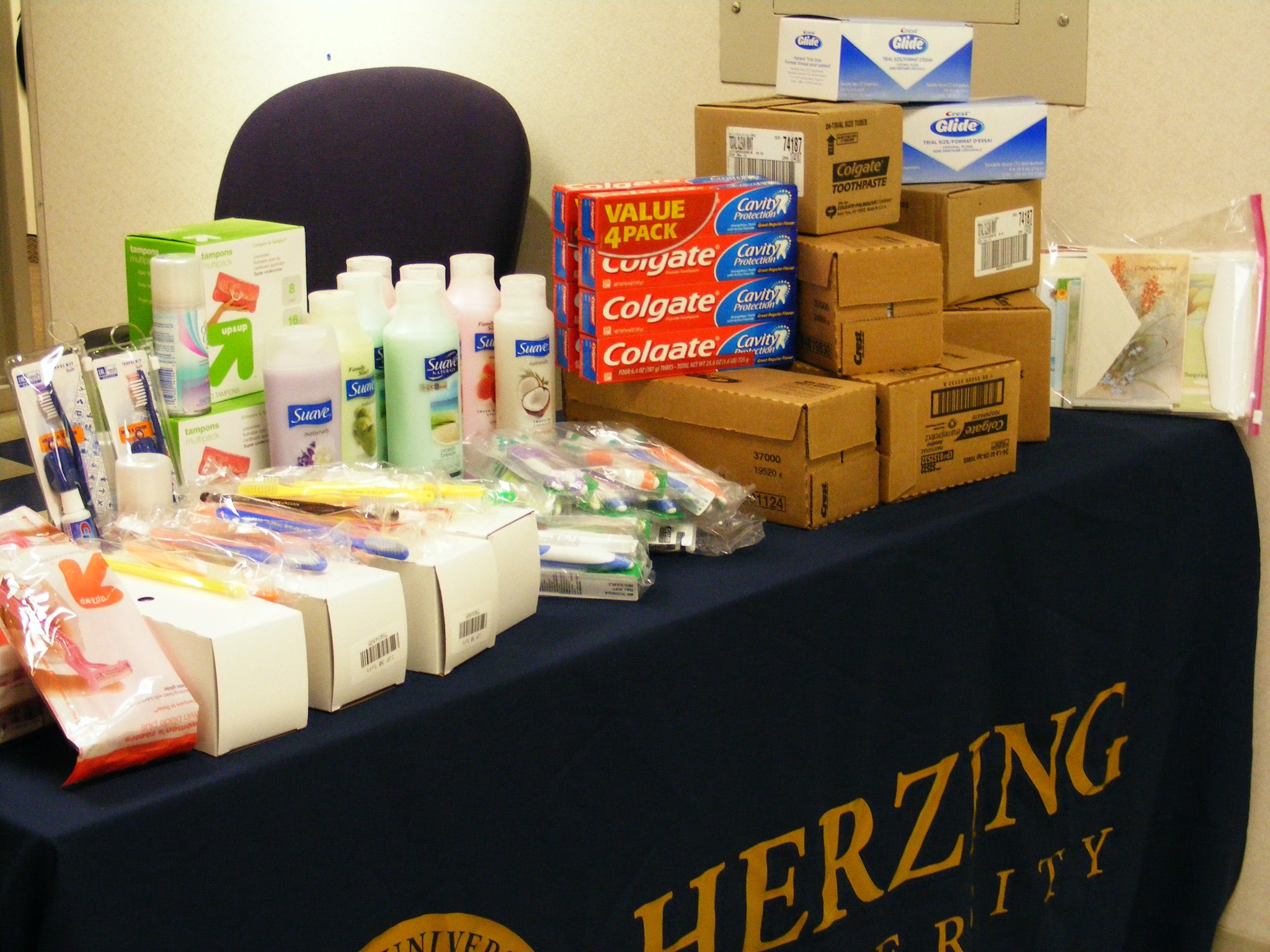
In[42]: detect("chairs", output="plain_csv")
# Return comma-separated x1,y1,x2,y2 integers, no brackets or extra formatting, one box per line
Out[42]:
212,62,534,276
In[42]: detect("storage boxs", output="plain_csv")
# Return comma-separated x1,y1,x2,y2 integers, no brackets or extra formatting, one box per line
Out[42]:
550,12,1061,529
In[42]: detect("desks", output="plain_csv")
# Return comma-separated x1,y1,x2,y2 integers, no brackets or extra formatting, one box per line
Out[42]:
0,404,1249,952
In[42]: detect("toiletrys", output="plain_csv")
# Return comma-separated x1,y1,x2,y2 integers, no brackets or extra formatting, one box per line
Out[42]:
547,176,802,389
259,249,560,488
59,487,106,566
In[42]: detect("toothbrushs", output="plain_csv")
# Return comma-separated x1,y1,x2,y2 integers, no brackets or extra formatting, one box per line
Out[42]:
36,381,97,521
124,365,176,485
113,411,754,603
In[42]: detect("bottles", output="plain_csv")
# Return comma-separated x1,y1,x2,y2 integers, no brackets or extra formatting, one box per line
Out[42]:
262,254,558,482
